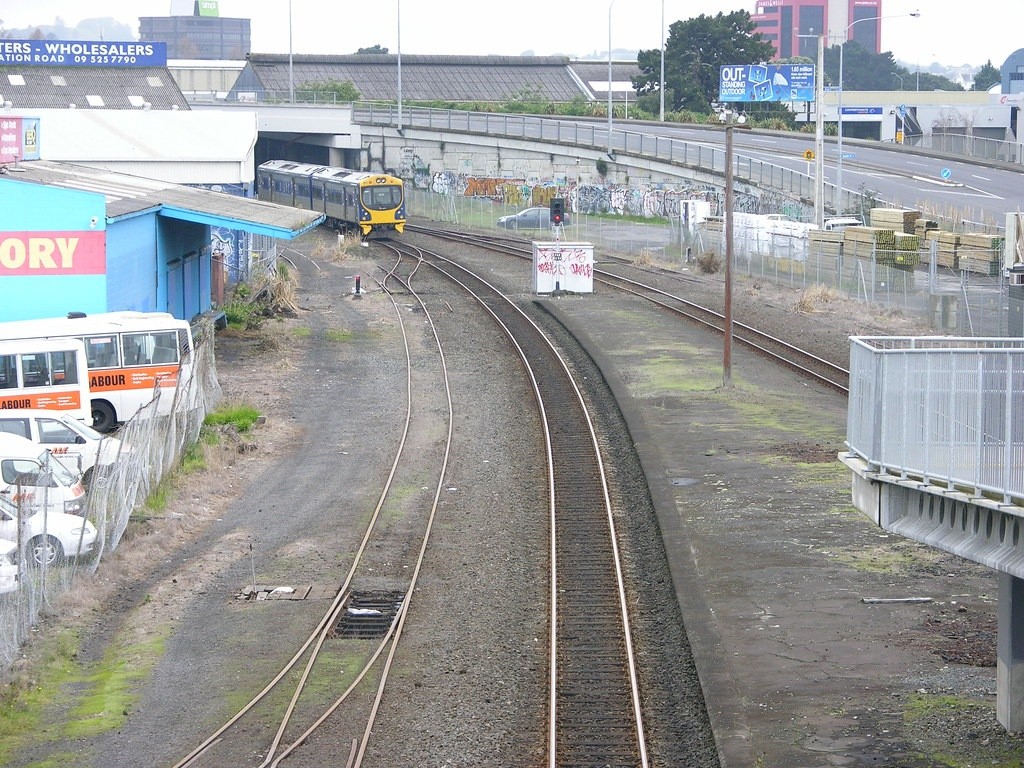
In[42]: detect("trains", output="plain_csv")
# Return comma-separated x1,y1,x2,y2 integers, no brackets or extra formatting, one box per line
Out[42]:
257,159,406,240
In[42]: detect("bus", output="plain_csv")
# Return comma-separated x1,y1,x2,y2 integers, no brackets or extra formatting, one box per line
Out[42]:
0,311,197,432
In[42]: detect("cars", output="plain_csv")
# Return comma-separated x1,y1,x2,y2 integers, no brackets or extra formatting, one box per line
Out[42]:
0,494,98,569
497,205,571,231
0,540,28,594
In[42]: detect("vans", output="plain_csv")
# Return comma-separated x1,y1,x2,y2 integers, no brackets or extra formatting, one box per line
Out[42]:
0,432,88,518
0,408,136,496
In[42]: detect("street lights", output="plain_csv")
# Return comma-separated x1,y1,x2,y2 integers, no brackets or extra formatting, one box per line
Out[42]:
835,10,920,217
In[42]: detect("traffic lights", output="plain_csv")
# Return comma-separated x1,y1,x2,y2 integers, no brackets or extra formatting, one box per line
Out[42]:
550,197,564,223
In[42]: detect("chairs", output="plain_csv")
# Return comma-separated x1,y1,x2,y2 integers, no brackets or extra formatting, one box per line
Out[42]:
7,361,77,389
94,341,175,367
